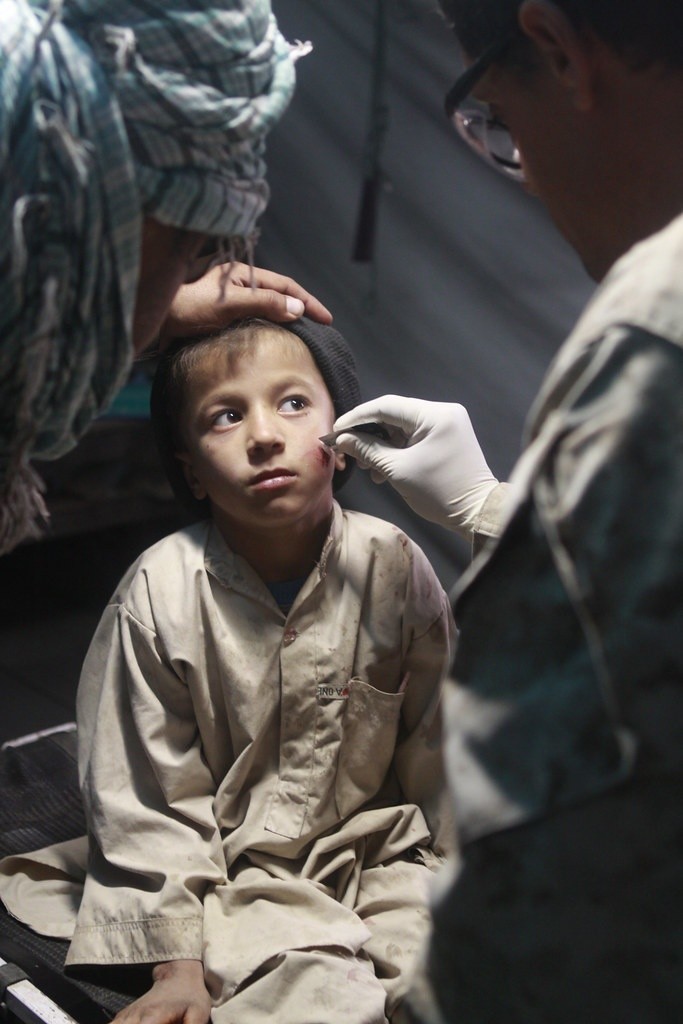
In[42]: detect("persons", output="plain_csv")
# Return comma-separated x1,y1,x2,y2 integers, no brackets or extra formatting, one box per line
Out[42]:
0,0,683,1024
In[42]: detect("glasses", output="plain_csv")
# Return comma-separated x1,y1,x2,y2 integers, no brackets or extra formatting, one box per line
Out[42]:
444,26,528,184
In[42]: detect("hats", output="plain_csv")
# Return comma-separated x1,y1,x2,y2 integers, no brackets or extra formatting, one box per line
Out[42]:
263,311,362,491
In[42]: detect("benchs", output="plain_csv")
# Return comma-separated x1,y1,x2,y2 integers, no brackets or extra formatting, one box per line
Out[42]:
18,460,186,563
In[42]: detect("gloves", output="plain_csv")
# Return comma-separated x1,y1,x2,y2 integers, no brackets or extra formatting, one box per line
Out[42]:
332,393,501,544
